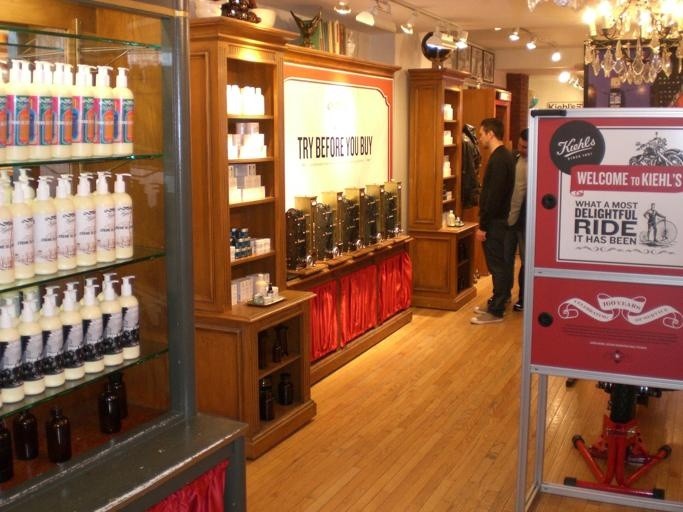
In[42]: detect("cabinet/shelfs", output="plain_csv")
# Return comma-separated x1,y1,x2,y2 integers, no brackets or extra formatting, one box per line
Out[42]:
408,69,480,309
0,1,250,512
187,15,317,461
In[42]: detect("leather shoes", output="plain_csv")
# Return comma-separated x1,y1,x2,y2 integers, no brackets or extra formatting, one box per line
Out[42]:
474,306,507,316
470,310,505,325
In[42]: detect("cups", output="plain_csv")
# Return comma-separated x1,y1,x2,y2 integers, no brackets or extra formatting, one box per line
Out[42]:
228,122,268,160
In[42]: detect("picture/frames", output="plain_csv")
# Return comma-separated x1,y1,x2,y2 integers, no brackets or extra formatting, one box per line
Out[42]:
451,41,495,83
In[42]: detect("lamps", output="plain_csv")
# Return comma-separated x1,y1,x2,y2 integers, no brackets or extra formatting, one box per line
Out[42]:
509,0,683,90
333,0,469,49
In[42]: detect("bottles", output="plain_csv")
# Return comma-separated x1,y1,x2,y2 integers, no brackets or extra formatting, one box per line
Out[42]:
230,226,271,260
445,103,455,121
227,84,267,117
43,406,73,465
444,209,456,226
99,374,128,435
259,326,295,420
1,411,39,485
254,273,274,304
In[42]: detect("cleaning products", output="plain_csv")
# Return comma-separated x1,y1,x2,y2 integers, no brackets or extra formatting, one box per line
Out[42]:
0,272,141,404
1,59,135,161
0,168,134,286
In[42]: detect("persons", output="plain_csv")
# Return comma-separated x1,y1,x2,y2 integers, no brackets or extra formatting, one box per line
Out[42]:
507,128,529,310
472,118,515,325
644,203,665,241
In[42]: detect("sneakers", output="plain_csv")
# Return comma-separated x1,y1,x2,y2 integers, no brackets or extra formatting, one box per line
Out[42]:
513,298,524,311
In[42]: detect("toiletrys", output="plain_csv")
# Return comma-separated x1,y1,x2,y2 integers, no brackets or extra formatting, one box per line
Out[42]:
228,85,278,303
